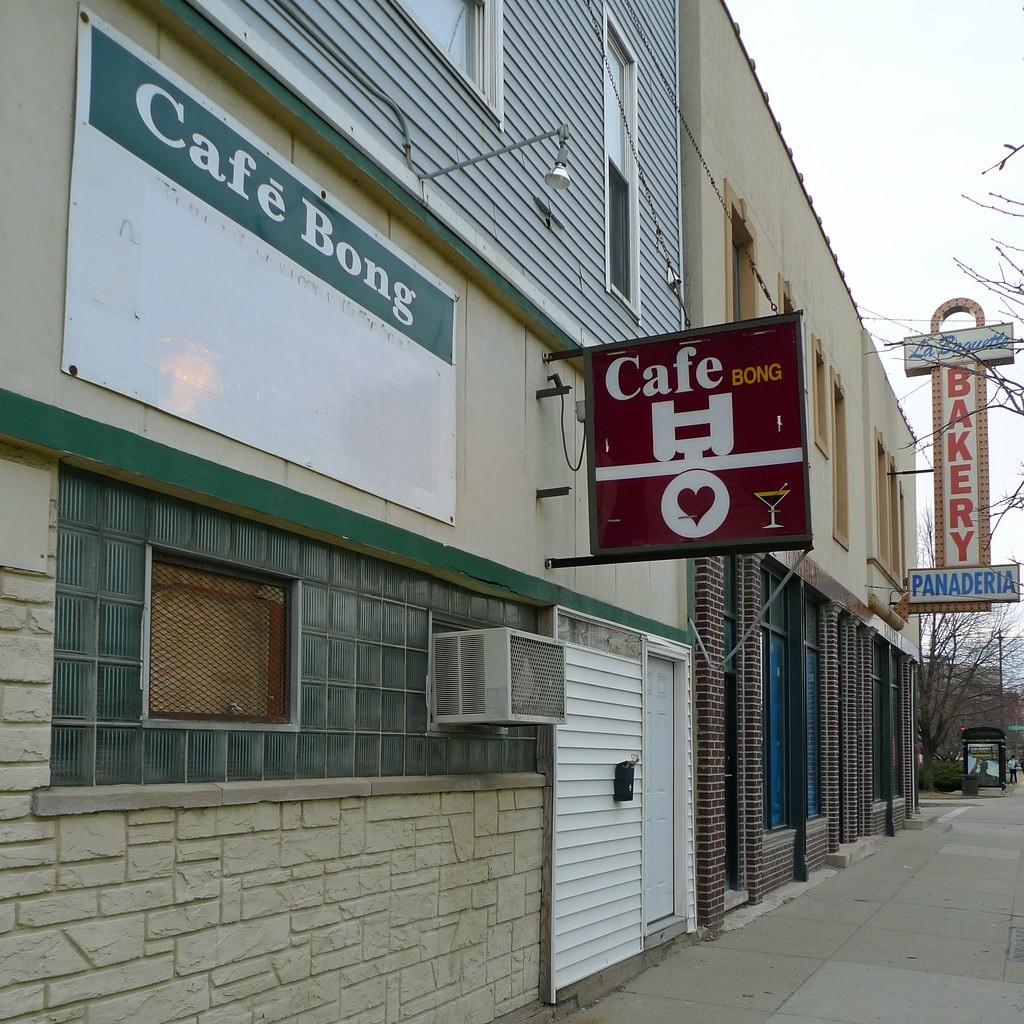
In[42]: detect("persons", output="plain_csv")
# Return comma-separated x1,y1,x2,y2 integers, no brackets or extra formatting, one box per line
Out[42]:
1021,761,1024,773
1007,754,1018,785
944,750,964,762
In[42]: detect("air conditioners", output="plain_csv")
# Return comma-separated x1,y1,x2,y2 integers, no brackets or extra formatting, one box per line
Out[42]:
431,627,568,726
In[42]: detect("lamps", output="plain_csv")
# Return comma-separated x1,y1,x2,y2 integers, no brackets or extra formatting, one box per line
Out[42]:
420,122,572,189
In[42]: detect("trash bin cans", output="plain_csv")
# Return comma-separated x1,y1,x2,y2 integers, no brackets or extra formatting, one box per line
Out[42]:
959,775,979,796
